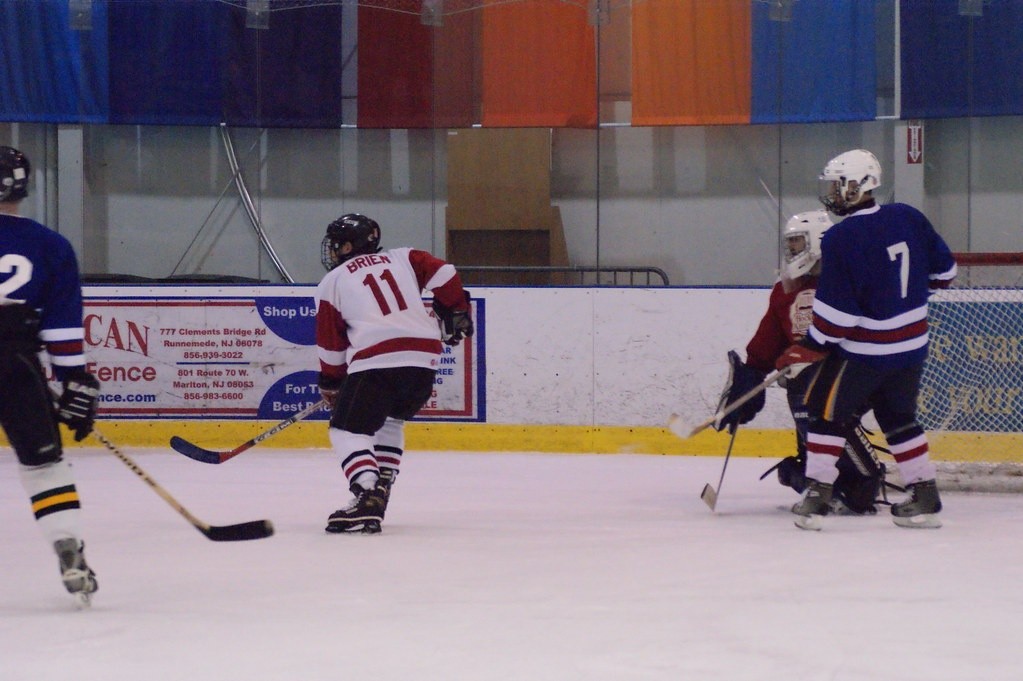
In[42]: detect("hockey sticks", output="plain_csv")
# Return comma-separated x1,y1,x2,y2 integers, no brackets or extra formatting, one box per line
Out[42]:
666,367,791,440
699,423,740,513
50,386,275,542
169,393,340,465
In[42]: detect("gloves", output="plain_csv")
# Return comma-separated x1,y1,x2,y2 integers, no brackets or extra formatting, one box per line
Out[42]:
317,370,344,404
432,290,474,348
774,341,833,379
57,366,100,443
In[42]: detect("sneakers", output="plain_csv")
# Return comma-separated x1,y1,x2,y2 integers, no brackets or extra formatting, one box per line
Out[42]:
890,478,942,527
326,468,394,533
791,479,833,532
54,537,98,609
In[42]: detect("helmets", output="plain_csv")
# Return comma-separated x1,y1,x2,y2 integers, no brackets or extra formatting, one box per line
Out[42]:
820,149,882,215
321,214,381,273
0,145,30,202
782,209,835,280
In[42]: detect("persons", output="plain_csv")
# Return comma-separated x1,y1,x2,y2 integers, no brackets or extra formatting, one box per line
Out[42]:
315,212,473,533
789,150,957,526
714,211,883,516
1,147,100,604
